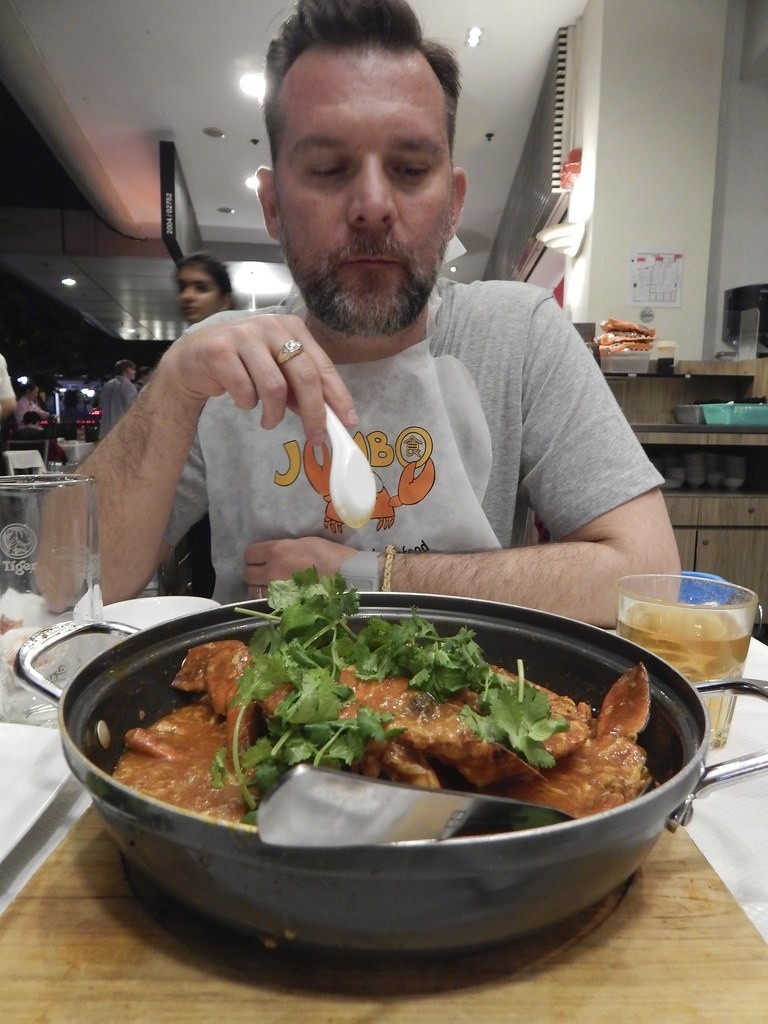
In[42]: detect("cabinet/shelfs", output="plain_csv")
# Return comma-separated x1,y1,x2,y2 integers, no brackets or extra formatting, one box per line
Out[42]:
630,423,768,624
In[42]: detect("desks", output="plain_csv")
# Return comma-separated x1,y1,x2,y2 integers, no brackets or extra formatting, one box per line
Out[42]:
59,442,94,463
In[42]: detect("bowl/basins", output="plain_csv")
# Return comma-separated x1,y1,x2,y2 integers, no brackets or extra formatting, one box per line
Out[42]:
648,451,746,490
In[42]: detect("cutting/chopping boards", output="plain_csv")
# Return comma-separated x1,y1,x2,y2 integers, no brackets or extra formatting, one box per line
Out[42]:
0,802,768,1024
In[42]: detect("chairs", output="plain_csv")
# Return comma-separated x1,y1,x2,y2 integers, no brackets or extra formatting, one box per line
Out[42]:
4,450,46,476
4,439,49,465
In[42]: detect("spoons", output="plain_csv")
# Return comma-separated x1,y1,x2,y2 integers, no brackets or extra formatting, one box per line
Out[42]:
323,401,378,531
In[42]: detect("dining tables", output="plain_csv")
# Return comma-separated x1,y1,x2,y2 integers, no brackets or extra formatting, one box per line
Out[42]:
0,628,767,1024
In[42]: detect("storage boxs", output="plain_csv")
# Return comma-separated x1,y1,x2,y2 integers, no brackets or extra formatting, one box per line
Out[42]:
600,347,651,374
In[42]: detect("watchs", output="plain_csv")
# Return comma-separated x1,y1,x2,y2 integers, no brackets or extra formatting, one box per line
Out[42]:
337,551,381,592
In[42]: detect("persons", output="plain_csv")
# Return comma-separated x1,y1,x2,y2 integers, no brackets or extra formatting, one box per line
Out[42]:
173,255,234,326
98,359,153,440
14,383,50,429
0,353,17,425
9,411,49,451
33,0,682,628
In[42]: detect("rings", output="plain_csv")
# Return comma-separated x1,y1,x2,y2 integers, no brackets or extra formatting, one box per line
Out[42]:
276,339,305,365
255,586,263,599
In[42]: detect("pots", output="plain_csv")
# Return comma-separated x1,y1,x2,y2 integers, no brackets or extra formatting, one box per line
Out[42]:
14,587,768,957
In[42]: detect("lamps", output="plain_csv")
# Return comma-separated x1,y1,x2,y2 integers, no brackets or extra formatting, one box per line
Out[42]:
535,223,585,258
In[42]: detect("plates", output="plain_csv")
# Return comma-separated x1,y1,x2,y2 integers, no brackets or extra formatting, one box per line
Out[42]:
0,726,74,863
101,594,220,632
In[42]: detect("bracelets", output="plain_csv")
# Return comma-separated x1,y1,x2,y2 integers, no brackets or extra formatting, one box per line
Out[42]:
381,545,396,592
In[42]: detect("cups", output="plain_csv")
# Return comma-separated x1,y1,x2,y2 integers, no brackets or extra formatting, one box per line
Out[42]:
1,473,103,724
614,573,758,750
656,339,679,376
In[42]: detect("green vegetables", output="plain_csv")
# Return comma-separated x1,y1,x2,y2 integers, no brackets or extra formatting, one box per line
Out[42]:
208,564,570,826
